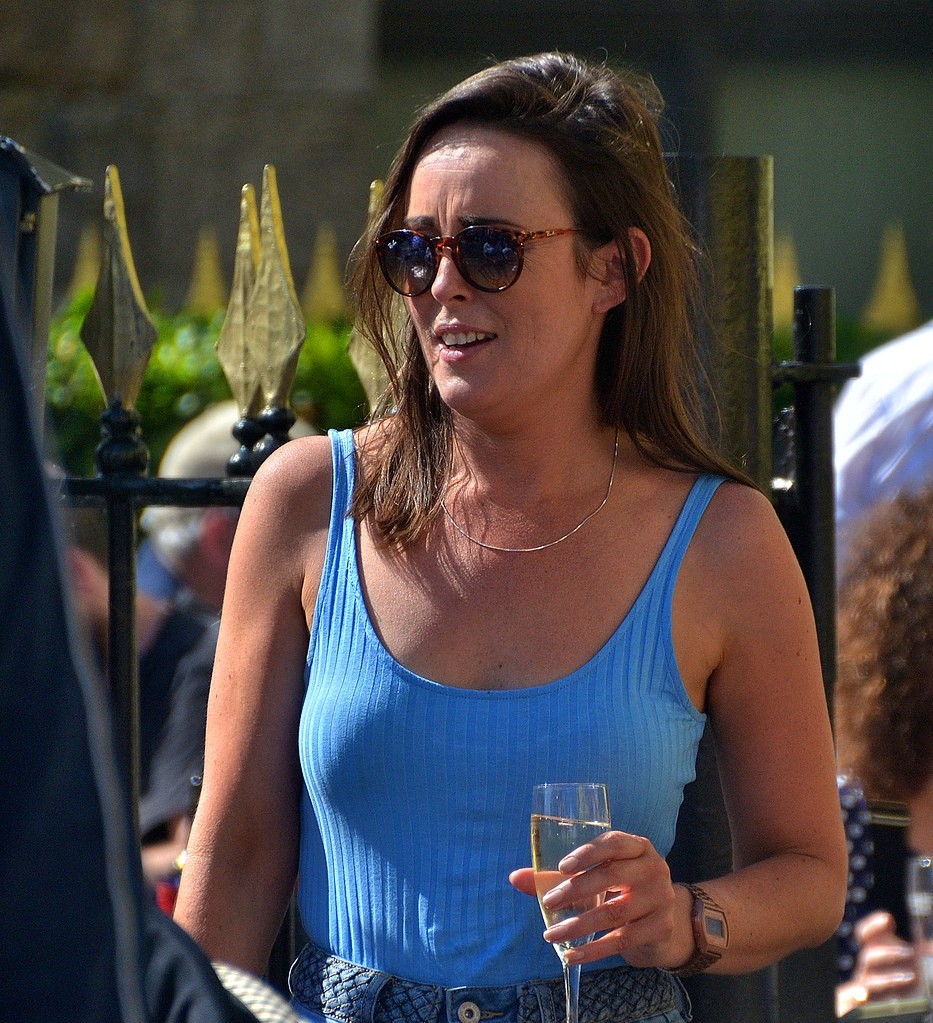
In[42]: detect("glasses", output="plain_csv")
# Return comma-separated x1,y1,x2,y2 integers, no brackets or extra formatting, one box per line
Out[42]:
372,227,595,298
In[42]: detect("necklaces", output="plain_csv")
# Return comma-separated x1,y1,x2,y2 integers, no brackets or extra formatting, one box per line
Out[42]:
432,425,620,551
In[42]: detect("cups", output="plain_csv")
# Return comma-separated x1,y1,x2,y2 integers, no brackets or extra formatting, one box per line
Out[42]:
906,859,933,1003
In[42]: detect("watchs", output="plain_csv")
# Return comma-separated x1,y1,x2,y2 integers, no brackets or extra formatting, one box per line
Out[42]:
657,881,731,978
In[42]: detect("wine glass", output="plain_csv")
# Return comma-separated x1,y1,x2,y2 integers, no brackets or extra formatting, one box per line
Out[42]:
529,783,612,1023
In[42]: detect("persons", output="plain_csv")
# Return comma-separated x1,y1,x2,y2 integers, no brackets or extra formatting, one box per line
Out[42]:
64,398,320,887
773,317,933,1023
171,52,847,1023
